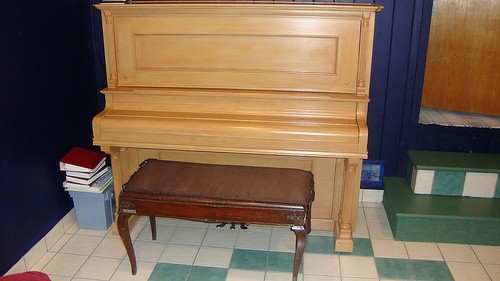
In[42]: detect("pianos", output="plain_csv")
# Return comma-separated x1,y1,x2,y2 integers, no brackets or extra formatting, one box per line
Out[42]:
92,86,370,253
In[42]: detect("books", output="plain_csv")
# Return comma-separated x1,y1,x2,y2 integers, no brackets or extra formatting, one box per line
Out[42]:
58,147,113,194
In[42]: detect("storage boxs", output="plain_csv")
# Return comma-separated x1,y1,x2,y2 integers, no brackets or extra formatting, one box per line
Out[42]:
69,180,117,230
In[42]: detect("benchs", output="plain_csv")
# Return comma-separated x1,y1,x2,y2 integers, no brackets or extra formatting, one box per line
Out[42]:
116,159,315,281
